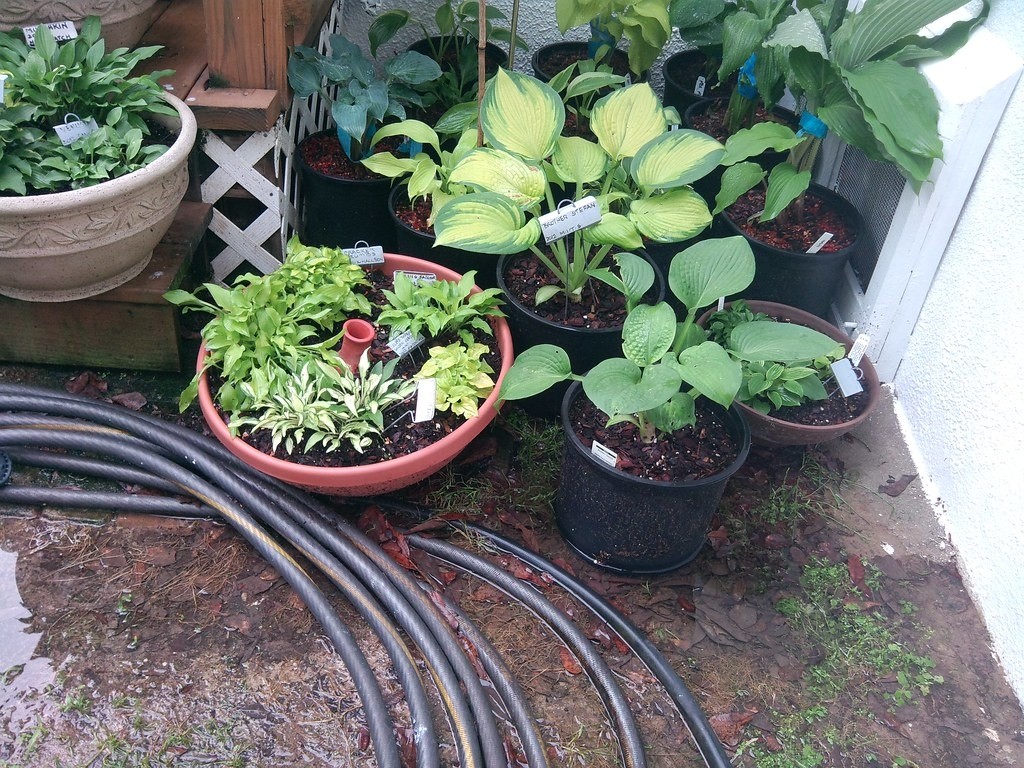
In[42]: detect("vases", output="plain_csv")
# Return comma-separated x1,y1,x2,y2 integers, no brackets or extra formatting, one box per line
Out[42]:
0,0,157,55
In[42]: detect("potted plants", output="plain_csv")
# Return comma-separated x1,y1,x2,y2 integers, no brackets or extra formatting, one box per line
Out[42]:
684,1,839,207
529,0,671,101
695,297,882,447
431,67,725,420
287,10,441,249
661,1,786,112
540,68,622,211
401,0,527,114
0,17,197,304
716,1,994,323
499,235,752,577
164,249,514,494
385,119,497,274
